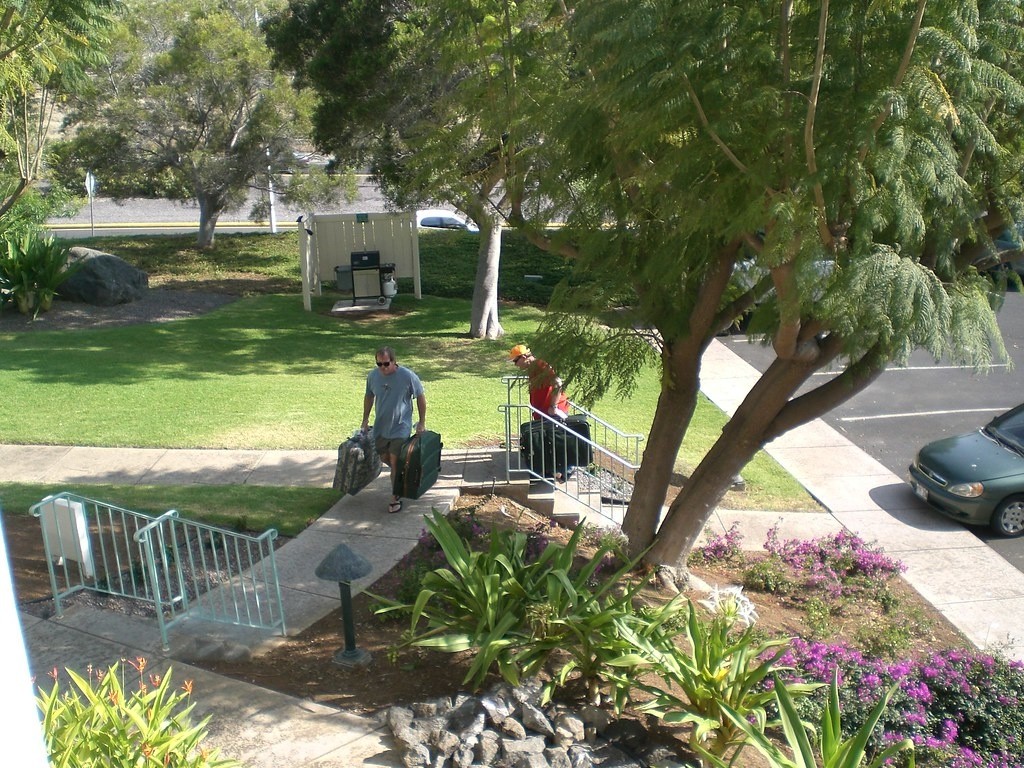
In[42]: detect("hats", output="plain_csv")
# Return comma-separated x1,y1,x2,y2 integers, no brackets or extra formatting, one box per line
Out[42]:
509,345,530,361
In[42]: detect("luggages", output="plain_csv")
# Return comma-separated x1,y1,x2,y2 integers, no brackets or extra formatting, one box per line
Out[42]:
333,426,382,496
393,430,443,500
519,414,593,472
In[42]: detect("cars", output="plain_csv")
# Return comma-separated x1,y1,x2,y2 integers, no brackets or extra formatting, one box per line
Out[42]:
715,258,838,339
909,403,1024,538
412,208,479,232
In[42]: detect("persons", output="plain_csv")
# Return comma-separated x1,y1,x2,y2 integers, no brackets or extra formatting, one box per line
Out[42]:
358,346,426,513
507,345,575,482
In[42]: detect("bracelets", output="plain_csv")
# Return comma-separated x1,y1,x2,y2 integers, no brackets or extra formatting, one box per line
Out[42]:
549,401,558,410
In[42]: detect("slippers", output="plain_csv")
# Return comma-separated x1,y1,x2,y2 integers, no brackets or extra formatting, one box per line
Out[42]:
559,470,575,484
388,500,402,513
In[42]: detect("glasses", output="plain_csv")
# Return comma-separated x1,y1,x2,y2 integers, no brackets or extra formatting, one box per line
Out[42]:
513,357,521,363
375,360,393,367
